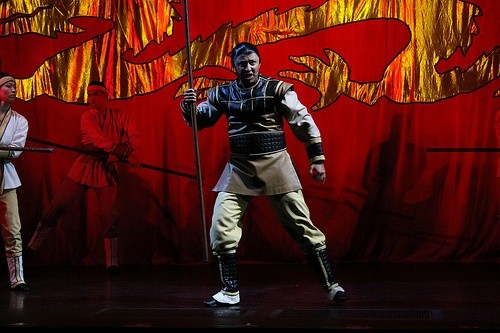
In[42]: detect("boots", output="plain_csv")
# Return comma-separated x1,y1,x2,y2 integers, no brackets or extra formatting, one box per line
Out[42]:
23,223,50,260
103,236,118,274
6,255,30,291
201,249,240,307
310,243,350,302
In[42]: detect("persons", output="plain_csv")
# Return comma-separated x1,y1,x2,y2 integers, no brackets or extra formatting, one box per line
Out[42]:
0,71,31,290
181,41,351,308
22,79,136,278
